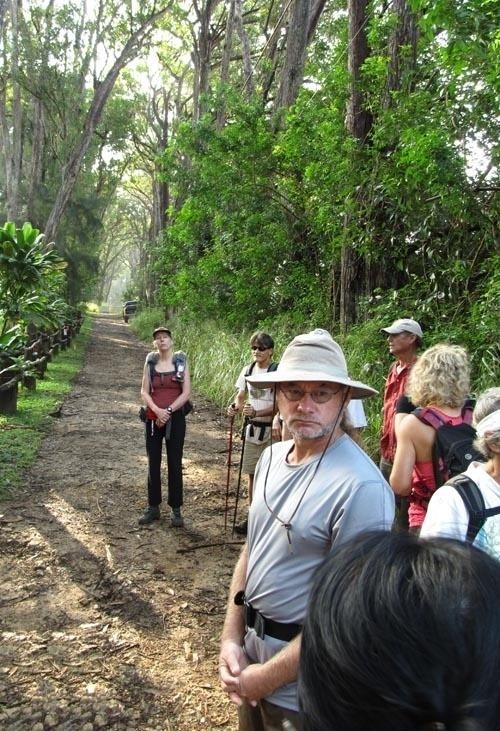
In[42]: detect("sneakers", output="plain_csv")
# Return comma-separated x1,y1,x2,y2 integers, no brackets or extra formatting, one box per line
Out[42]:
171,506,183,526
139,506,160,524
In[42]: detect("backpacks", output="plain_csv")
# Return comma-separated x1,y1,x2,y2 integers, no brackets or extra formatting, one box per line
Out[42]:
409,399,488,489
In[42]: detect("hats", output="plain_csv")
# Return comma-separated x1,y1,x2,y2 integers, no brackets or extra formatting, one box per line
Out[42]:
153,327,170,338
245,329,379,400
380,319,422,338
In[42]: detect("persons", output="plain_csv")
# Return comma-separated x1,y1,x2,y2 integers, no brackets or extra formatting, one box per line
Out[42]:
227,330,280,534
136,327,192,528
296,528,500,731
378,319,424,532
217,328,396,731
417,386,500,561
389,343,480,537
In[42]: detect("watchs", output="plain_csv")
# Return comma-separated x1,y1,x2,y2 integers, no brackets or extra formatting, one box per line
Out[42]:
167,407,172,413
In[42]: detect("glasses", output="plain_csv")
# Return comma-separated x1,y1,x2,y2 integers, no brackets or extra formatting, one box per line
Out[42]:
252,346,273,351
279,385,342,403
